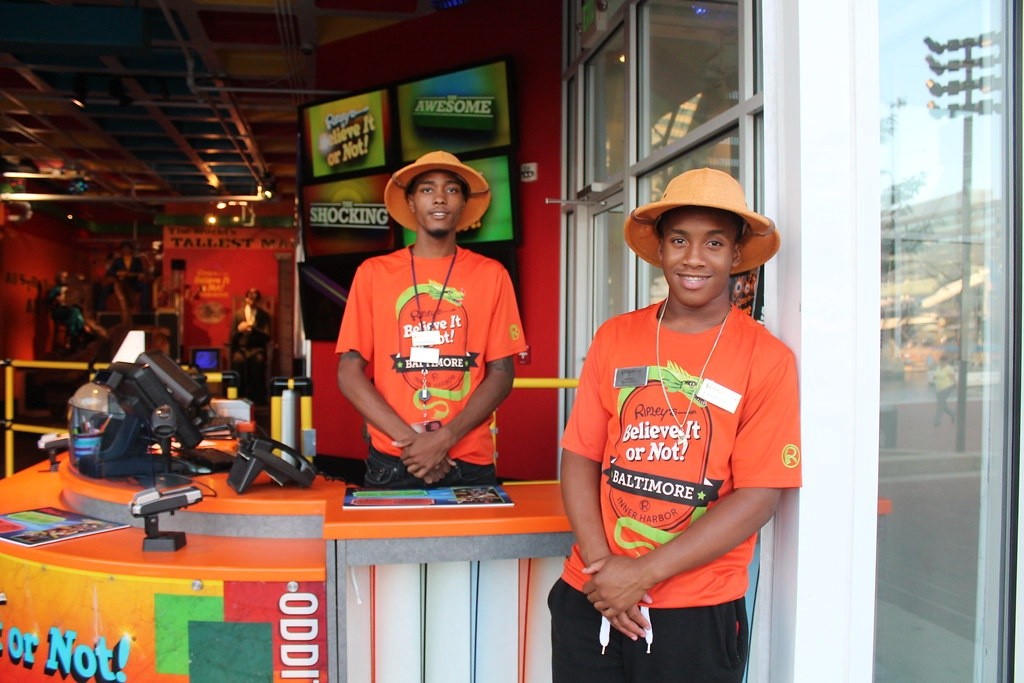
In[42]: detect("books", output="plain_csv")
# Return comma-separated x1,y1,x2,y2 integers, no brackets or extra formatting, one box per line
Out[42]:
0,507,131,549
342,488,514,509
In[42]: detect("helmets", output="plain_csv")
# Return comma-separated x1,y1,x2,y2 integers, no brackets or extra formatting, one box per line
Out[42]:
624,167,781,275
383,150,491,231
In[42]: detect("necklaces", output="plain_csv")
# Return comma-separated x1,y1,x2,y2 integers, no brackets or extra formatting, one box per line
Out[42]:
657,298,730,440
411,247,457,403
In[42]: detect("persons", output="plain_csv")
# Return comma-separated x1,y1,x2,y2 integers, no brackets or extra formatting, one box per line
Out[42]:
335,151,527,489
45,271,92,348
106,241,151,312
547,168,802,683
929,356,958,429
229,288,270,406
